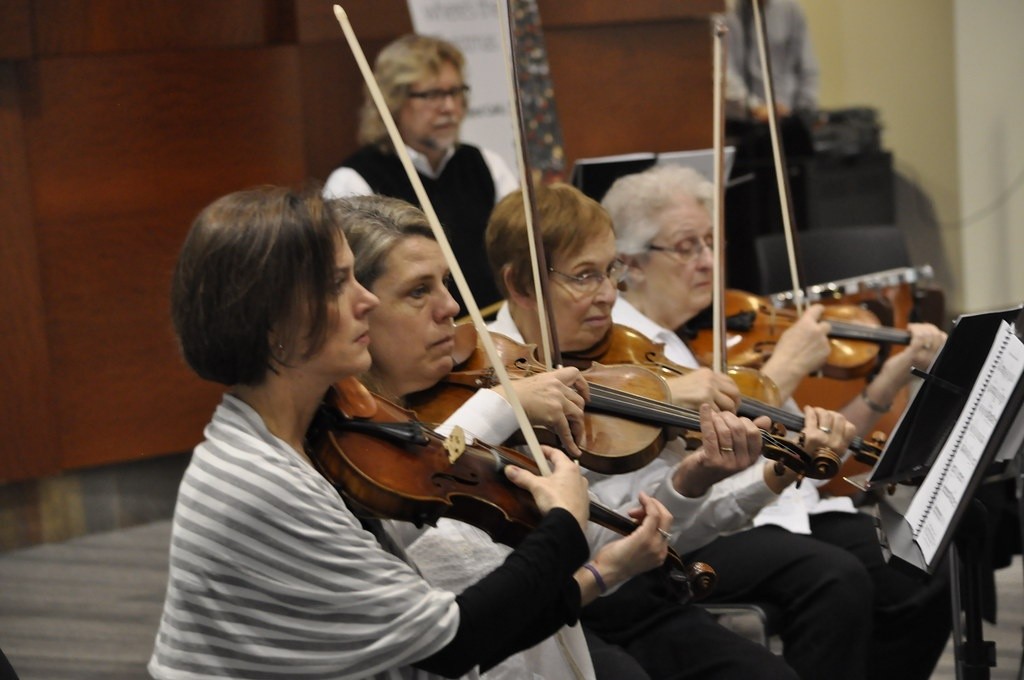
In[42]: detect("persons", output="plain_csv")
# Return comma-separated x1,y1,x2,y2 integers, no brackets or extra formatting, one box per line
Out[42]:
145,168,947,680
324,34,518,325
721,0,827,232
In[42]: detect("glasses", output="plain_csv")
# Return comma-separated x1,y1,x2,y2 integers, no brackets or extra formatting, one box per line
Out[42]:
649,243,714,262
409,85,470,106
548,257,631,293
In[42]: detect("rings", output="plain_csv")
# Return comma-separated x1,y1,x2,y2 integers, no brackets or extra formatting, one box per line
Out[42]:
720,446,734,451
660,530,671,540
820,427,831,434
923,345,931,349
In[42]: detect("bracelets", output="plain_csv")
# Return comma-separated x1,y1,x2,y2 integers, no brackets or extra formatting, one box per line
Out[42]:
583,563,607,597
861,388,892,414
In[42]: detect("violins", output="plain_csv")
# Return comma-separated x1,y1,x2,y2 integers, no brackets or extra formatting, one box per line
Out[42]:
560,323,887,467
305,376,717,598
673,289,913,381
402,322,842,480
